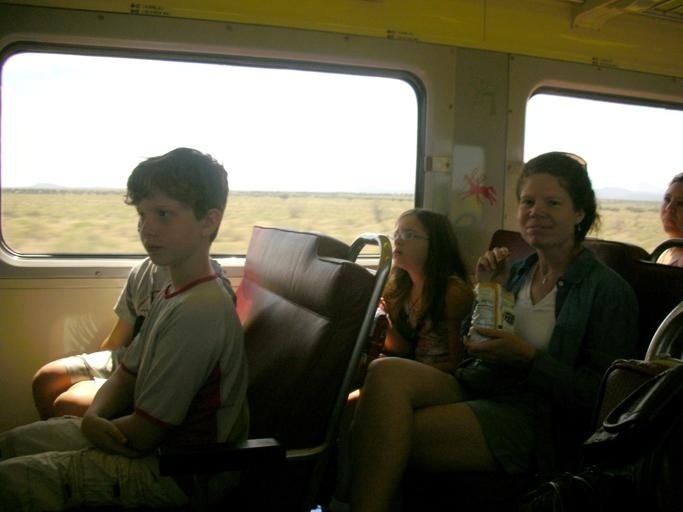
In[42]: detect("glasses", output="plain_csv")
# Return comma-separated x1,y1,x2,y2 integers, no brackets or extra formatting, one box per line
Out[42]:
389,231,430,242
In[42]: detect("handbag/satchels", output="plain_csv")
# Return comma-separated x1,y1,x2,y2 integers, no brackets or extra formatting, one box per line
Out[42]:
518,468,636,511
583,364,683,465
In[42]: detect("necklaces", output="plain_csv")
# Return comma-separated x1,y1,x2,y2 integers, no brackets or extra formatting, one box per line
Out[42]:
542,273,548,285
400,291,421,322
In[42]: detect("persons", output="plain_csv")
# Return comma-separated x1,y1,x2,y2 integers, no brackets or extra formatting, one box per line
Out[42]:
29,252,238,420
660,176,683,267
327,207,474,512
319,151,642,512
0,147,251,512
